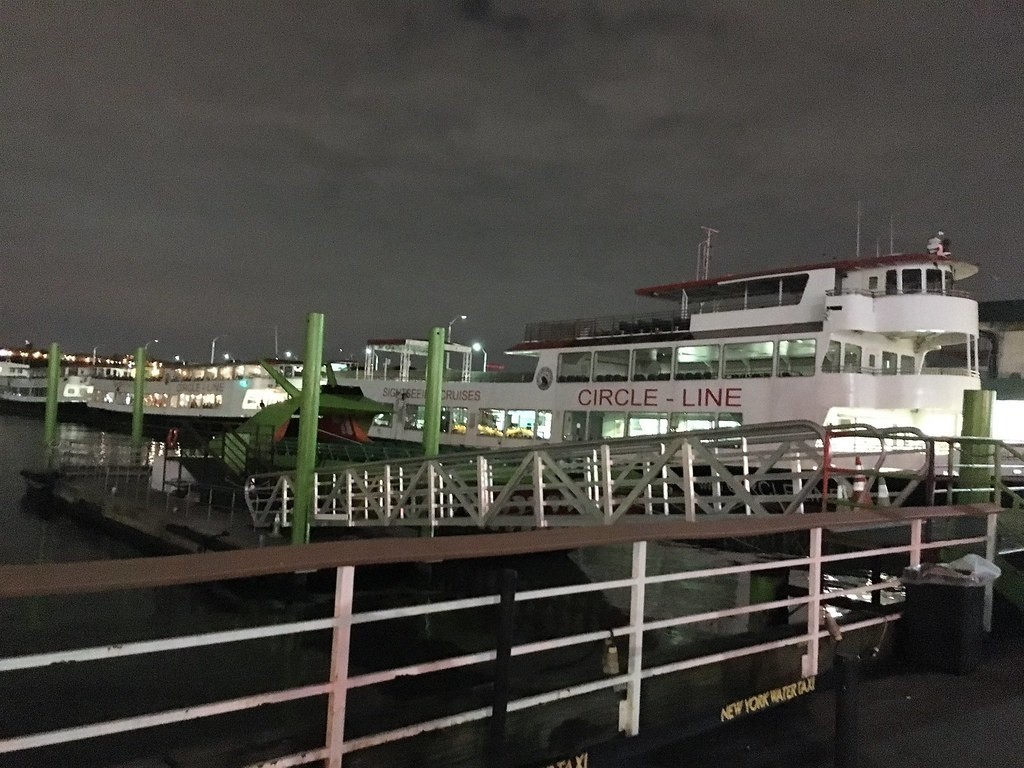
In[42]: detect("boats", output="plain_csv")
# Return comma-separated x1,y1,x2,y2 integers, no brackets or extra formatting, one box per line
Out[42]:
0,360,91,407
165,361,538,520
332,196,1024,514
86,358,355,428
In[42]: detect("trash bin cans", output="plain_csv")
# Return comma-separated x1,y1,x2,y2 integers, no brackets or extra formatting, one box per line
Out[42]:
902,554,1002,676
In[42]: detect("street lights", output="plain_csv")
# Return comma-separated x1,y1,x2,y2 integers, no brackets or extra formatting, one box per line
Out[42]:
210,334,228,364
224,354,235,363
145,340,159,355
365,347,378,371
175,355,185,365
473,342,487,373
445,315,466,368
286,351,297,361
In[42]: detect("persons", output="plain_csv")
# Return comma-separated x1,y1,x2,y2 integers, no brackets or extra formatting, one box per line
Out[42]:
260,400,265,409
346,364,356,371
191,400,197,407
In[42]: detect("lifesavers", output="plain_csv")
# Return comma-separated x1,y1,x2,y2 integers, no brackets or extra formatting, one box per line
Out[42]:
166,426,178,449
